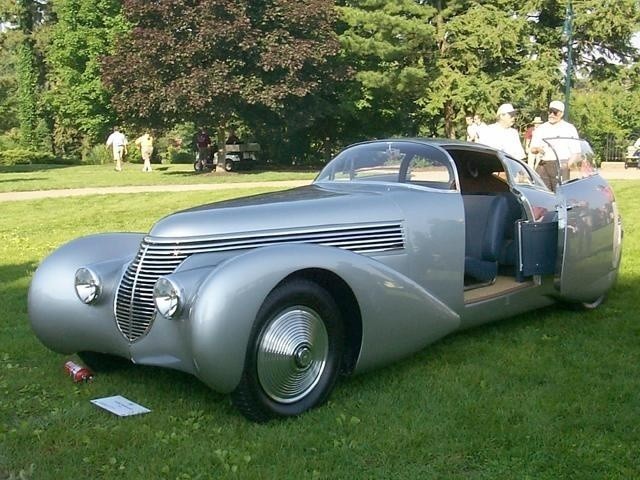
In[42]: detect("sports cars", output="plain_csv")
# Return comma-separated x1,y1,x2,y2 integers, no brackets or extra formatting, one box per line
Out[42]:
23,132,624,426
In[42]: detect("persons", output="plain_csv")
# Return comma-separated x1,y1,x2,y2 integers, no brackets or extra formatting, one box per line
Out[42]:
515,148,623,291
225,129,239,145
195,125,212,173
478,103,528,186
464,115,478,141
527,100,582,195
524,116,543,170
106,126,128,172
134,128,154,172
473,112,487,144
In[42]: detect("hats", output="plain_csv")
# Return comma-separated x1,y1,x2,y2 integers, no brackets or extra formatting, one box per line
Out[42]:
497,103,518,115
532,116,543,124
549,101,564,112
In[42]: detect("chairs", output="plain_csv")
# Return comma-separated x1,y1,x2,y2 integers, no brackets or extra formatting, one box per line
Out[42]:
462,189,524,285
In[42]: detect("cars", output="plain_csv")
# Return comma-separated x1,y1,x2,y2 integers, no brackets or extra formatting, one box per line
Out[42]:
624,150,640,170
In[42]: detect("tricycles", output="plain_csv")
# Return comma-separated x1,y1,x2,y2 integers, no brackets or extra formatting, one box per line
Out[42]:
193,139,260,174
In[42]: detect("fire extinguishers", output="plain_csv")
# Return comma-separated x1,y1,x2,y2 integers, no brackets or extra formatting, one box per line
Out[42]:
64,361,93,383
